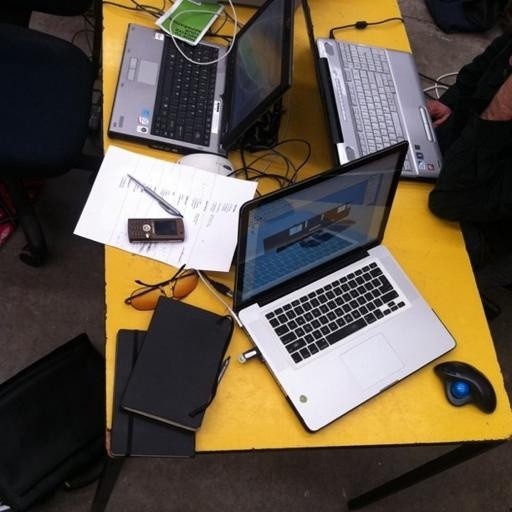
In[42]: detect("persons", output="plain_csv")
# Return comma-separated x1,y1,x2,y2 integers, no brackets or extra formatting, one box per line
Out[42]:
425,26,510,317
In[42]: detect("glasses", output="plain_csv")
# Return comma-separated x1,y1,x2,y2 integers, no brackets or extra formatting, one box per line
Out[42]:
124,263,199,310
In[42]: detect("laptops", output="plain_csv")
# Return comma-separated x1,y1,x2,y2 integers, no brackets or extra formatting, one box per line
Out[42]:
302,0,444,181
108,0,295,158
231,141,457,433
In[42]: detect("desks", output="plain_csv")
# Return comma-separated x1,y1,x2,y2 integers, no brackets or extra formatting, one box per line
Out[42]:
91,1,511,511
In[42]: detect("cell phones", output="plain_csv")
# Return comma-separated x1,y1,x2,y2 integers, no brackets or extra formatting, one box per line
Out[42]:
127,219,186,244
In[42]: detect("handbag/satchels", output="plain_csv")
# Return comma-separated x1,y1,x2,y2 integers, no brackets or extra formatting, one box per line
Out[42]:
0,333,106,512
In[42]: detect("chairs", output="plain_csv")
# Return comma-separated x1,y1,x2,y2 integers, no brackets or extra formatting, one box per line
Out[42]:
0,0,102,267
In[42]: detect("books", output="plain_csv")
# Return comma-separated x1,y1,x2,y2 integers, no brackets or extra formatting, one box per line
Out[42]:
122,295,234,432
110,329,198,458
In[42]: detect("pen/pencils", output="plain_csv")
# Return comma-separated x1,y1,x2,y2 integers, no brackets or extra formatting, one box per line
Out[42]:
218,355,231,383
143,186,183,218
